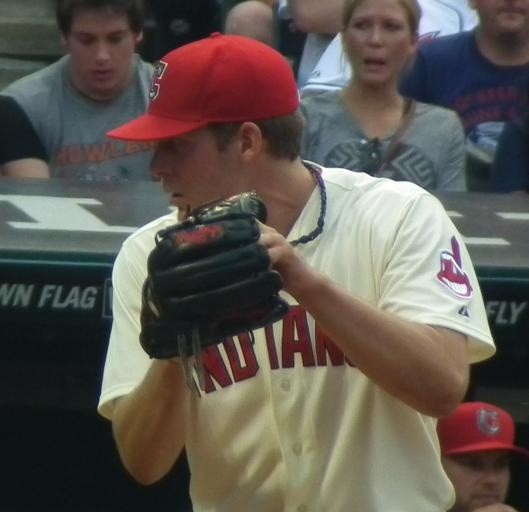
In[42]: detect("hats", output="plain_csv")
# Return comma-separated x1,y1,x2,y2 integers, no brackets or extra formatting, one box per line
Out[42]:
436,401,529,458
106,33,299,142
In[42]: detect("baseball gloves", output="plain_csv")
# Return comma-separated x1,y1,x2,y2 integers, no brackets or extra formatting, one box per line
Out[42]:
139,190,289,357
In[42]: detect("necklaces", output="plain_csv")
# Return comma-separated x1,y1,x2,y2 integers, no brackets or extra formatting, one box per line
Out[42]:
283,162,330,245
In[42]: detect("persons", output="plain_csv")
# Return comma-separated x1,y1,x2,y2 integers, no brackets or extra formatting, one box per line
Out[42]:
433,399,525,511
275,1,481,95
96,30,499,511
395,1,529,195
297,0,468,192
137,0,282,66
0,0,157,180
